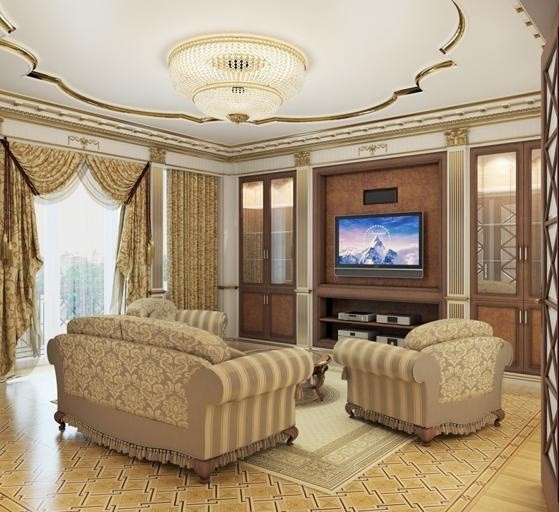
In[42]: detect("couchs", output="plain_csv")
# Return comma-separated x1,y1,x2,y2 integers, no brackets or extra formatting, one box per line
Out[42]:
46,315,304,488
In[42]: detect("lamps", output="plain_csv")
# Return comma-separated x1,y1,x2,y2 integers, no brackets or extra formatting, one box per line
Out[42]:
162,30,312,128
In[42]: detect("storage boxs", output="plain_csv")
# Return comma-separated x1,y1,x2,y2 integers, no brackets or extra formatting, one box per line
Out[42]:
337,327,374,349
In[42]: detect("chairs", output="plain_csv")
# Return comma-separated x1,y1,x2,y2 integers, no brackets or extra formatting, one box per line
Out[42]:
124,297,229,339
331,319,515,448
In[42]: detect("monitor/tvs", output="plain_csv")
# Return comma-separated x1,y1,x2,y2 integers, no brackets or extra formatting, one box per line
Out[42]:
333,211,424,279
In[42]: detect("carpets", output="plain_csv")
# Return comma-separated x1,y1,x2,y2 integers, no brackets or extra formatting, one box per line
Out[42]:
233,347,418,503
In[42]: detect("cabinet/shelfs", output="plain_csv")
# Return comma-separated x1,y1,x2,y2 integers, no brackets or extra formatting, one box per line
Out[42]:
470,139,542,379
238,169,297,345
314,286,446,353
